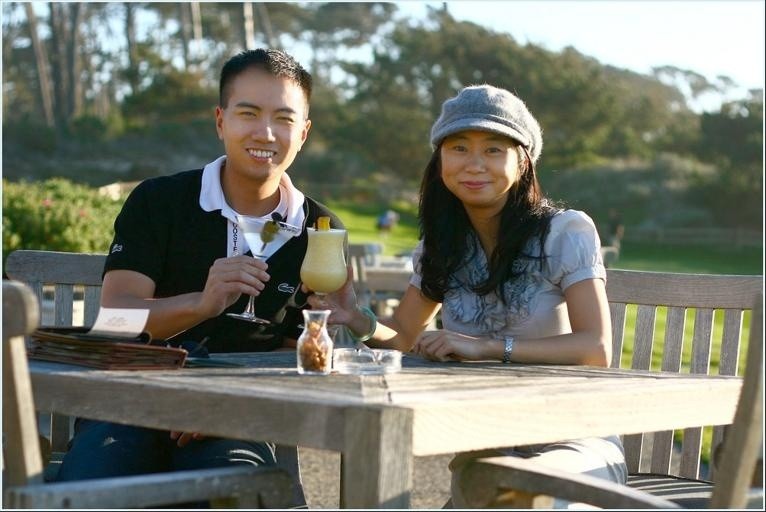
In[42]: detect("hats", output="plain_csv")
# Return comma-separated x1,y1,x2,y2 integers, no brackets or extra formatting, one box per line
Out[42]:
430,83,543,162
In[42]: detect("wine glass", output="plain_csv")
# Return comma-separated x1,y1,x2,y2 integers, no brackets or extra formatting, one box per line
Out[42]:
300,227,350,329
223,215,299,328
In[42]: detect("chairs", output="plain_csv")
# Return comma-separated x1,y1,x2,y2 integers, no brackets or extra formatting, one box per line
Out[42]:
343,238,415,311
462,268,765,512
5,250,106,471
2,281,291,512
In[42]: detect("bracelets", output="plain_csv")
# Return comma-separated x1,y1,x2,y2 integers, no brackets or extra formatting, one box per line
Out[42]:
502,335,513,364
345,306,376,342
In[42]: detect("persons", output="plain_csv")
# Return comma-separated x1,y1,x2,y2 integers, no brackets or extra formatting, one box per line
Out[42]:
374,206,398,238
607,207,625,251
56,49,349,509
300,84,629,509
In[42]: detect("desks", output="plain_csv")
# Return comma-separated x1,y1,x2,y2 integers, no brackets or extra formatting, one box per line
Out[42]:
26,337,744,510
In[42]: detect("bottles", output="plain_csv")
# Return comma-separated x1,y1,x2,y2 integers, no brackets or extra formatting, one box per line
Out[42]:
296,309,334,376
